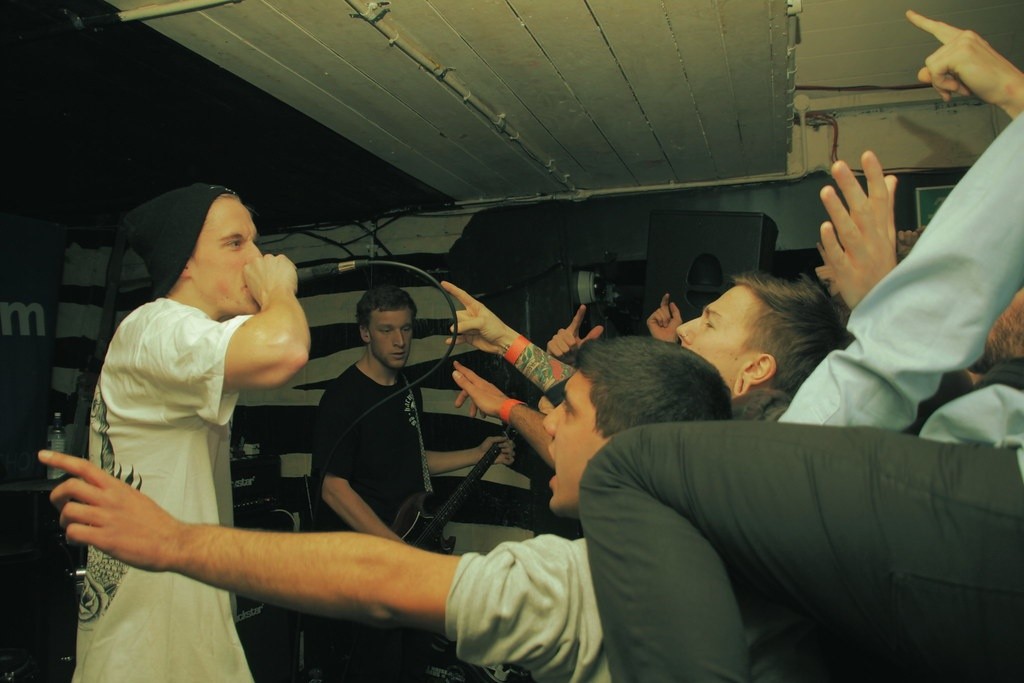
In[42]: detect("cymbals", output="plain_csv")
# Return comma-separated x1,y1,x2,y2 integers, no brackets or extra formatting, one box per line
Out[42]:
1,477,66,492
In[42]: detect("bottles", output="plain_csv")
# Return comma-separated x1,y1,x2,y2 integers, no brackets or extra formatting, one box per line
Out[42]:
46,412,68,481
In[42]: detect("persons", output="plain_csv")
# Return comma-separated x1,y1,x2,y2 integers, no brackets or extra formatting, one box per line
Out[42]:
38,10,1024,682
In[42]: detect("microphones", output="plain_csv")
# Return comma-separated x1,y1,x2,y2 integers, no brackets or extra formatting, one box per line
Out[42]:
296,260,371,286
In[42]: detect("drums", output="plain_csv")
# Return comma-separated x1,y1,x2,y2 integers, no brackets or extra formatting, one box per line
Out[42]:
0,646,34,683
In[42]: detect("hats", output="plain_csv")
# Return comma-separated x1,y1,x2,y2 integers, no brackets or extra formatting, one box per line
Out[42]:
124,182,236,299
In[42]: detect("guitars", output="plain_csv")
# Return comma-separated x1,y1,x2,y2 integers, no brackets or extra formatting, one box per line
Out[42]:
389,425,519,556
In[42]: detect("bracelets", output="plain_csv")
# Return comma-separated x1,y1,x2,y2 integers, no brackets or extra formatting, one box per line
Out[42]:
500,399,528,424
504,335,529,365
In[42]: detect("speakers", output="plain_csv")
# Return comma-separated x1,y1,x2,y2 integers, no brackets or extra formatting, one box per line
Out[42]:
640,209,779,338
41,494,300,683
